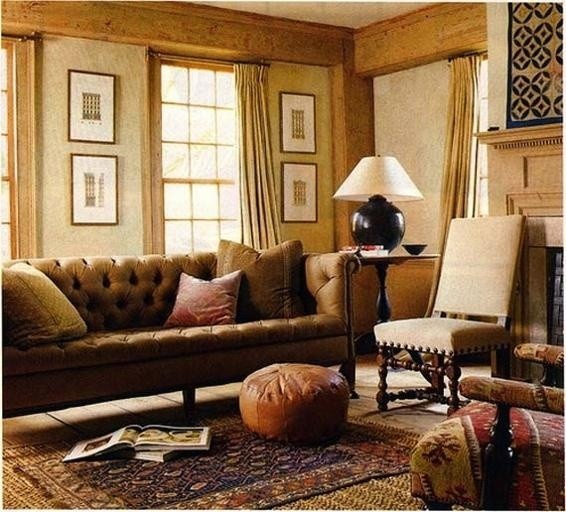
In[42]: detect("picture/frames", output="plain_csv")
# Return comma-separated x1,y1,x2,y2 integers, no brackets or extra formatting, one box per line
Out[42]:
280,161,318,223
67,68,116,145
70,153,119,226
278,90,317,154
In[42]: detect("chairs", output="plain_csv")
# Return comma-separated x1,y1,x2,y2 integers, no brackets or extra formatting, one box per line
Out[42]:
372,212,528,417
408,341,564,510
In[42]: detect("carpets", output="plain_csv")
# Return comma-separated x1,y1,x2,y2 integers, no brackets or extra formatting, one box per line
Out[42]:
4,391,453,509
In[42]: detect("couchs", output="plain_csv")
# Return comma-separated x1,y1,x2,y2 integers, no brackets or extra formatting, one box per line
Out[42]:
2,247,365,424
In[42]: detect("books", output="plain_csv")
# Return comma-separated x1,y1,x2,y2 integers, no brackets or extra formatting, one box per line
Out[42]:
59,423,215,463
338,244,390,258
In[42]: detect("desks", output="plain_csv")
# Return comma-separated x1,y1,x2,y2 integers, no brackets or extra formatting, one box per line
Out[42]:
353,254,441,374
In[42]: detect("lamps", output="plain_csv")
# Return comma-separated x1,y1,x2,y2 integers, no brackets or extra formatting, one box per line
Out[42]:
331,152,426,255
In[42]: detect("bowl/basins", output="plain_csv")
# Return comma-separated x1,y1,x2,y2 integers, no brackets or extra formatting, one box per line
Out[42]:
401,244,427,256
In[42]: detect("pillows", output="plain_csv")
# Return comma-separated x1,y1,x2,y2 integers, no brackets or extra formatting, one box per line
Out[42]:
162,269,242,329
215,238,304,319
2,260,89,349
